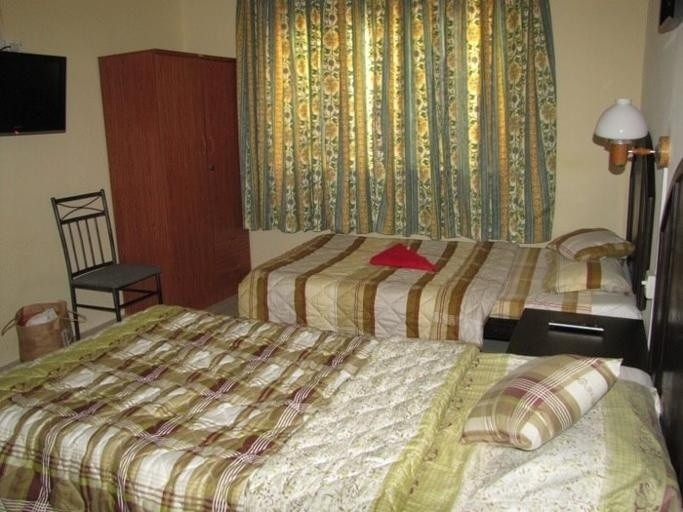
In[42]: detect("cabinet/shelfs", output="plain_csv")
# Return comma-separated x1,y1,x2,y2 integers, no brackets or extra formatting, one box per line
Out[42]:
97,49,251,316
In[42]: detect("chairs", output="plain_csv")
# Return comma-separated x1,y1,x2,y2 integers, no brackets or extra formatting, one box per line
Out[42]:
49,189,164,341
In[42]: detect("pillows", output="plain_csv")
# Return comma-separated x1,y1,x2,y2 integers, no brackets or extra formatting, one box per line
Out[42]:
545,252,633,295
545,227,637,262
459,353,624,452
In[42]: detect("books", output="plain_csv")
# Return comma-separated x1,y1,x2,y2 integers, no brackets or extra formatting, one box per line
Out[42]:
547,316,610,333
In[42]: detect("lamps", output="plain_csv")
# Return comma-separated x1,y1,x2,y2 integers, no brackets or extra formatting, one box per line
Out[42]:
592,97,670,174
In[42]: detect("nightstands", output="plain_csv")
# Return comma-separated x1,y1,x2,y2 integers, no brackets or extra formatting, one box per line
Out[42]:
506,308,649,369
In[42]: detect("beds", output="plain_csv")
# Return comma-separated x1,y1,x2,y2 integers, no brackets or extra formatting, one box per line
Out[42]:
0,303,682,511
239,233,638,344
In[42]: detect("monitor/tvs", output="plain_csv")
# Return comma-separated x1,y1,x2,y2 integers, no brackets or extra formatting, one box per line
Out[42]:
0,51,67,136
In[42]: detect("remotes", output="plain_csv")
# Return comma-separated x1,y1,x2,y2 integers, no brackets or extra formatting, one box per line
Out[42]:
547,320,605,336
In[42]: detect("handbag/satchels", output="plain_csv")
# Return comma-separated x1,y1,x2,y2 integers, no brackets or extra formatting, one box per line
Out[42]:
2,301,87,361
